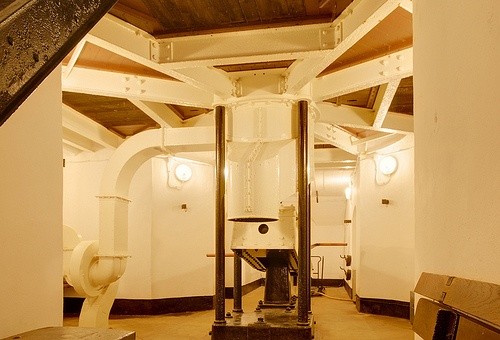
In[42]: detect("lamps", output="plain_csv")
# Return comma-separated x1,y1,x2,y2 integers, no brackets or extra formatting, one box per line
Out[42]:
174,162,193,182
378,154,398,175
